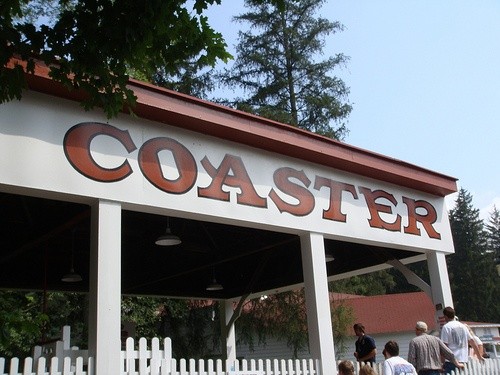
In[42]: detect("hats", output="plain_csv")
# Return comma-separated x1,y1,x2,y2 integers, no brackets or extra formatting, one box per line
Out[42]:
416,321,427,331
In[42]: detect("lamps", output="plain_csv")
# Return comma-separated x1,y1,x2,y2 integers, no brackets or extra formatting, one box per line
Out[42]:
206,265,224,291
62,228,83,282
155,216,182,246
325,240,335,262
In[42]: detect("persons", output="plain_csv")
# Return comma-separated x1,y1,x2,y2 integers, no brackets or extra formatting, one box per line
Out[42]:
337,307,489,375
